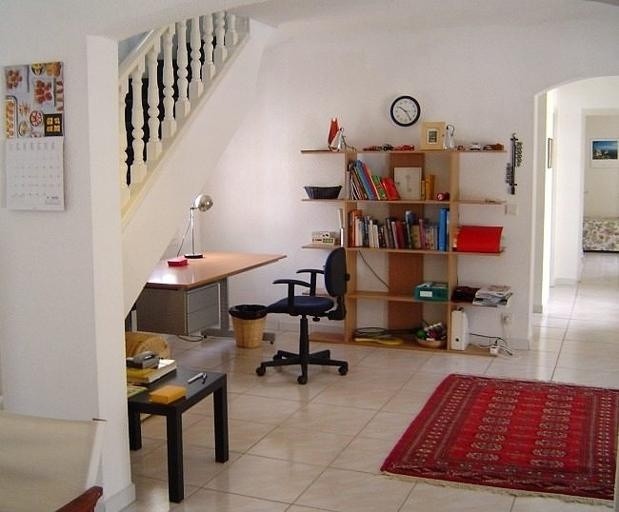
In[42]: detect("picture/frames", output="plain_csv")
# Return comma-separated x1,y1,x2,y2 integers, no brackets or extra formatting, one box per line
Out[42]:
419,121,446,151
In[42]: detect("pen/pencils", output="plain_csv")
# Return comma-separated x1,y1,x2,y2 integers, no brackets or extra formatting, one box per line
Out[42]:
187,372,207,384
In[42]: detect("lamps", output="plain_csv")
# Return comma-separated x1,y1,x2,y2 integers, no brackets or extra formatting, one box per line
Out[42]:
185,192,215,259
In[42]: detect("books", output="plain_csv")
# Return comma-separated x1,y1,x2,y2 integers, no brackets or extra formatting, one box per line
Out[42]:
454,224,506,254
127,359,178,385
344,157,450,252
148,384,189,404
472,283,514,307
128,384,147,400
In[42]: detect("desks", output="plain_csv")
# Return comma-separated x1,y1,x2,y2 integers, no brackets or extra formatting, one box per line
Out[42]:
124,364,228,504
135,249,287,352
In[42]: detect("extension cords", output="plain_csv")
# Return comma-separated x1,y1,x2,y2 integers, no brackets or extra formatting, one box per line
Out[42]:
489,343,500,355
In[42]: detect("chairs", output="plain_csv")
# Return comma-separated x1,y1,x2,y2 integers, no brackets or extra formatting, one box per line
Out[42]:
2,410,108,512
255,246,351,386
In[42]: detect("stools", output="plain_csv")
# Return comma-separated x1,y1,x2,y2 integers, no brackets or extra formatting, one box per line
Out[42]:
125,331,172,423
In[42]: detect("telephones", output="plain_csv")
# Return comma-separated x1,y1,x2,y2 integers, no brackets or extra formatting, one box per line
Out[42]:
126,350,160,369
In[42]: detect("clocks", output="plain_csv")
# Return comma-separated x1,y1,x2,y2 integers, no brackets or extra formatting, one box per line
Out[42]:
388,94,422,130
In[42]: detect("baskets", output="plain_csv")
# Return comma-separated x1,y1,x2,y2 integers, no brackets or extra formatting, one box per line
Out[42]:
414,335,447,348
305,185,342,199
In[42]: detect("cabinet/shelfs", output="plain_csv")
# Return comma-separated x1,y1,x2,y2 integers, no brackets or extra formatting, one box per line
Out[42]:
295,145,510,358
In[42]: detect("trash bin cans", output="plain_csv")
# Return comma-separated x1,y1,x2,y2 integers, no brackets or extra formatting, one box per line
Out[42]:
229,305,267,348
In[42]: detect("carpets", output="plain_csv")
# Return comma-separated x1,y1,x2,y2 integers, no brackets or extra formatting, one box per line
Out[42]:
379,372,618,507
